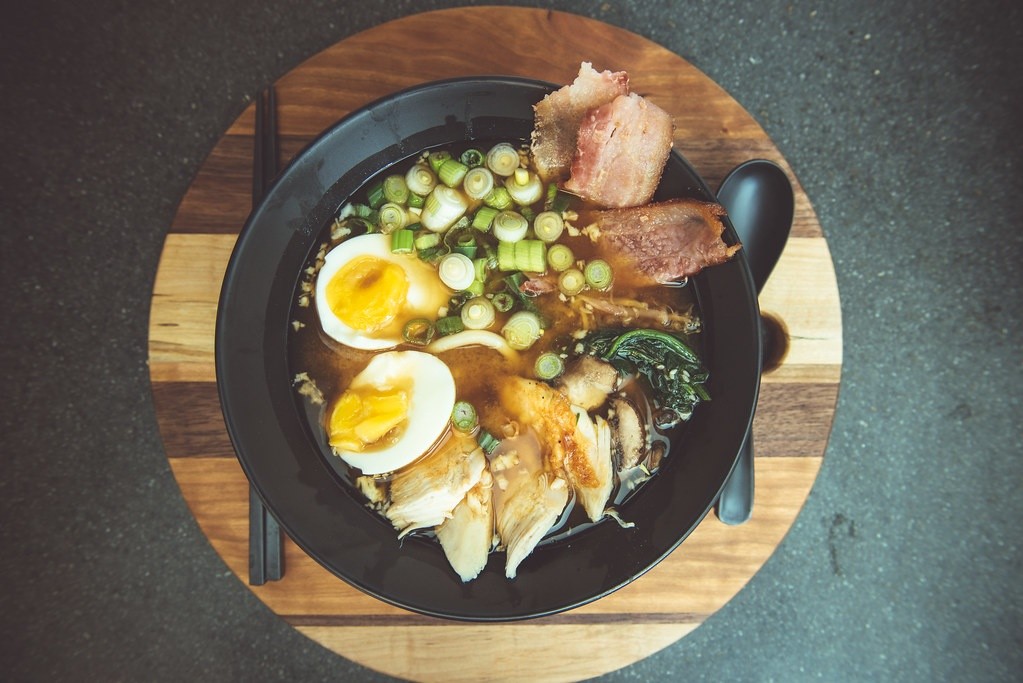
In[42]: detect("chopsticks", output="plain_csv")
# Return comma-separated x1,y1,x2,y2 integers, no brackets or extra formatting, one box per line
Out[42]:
241,82,288,592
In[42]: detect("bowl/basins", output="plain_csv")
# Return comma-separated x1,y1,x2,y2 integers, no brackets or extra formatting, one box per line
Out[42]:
215,69,766,628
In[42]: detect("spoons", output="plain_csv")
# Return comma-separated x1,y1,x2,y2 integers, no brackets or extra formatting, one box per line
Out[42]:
718,156,792,525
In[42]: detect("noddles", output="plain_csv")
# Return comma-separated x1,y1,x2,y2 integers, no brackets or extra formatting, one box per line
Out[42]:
215,58,760,622
